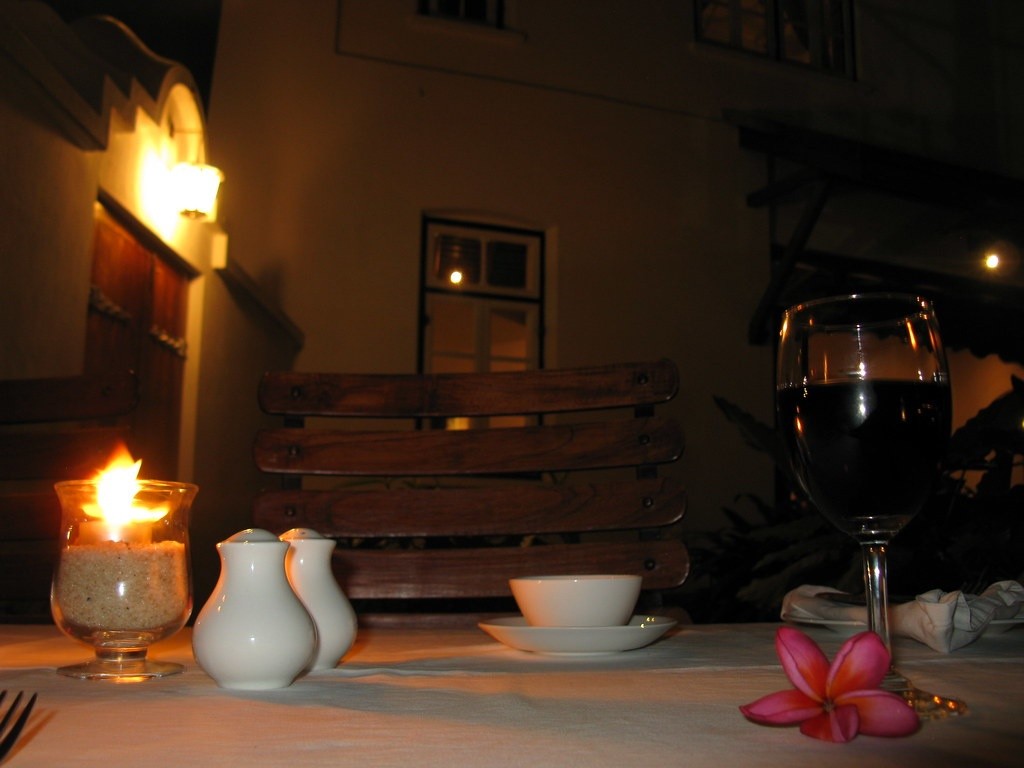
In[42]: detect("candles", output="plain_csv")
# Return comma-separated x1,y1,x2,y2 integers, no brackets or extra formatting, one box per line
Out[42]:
77,437,170,544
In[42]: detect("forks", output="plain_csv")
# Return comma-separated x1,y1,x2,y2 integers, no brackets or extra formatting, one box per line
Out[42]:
0,689,37,761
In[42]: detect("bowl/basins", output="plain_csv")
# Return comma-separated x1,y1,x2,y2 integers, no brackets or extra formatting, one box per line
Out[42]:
508,574,642,627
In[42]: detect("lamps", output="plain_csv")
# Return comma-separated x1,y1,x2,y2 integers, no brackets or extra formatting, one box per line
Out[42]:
167,163,226,220
450,271,461,284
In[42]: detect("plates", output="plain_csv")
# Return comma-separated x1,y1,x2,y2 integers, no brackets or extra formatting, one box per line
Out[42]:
478,616,678,650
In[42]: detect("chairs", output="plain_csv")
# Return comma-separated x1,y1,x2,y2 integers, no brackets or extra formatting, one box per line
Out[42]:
247,353,690,623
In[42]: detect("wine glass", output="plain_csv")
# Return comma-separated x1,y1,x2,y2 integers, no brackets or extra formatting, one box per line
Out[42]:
772,290,971,724
